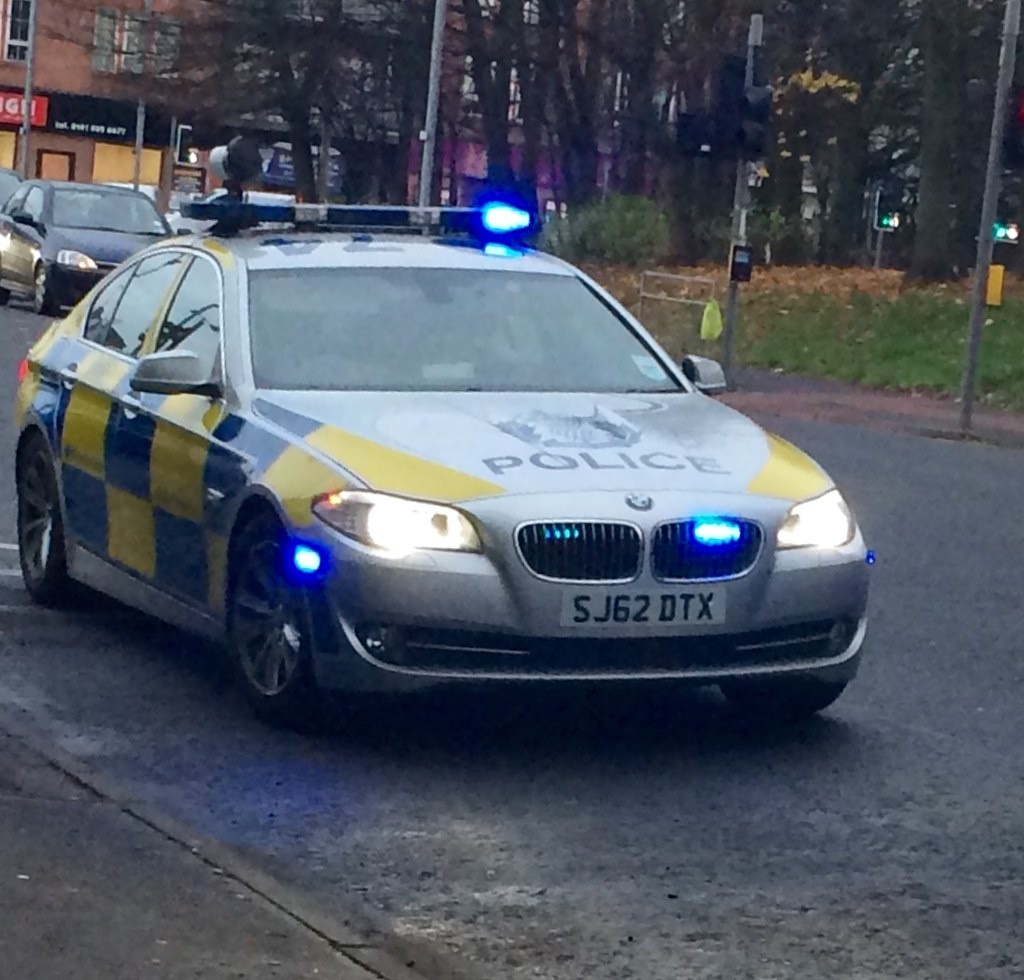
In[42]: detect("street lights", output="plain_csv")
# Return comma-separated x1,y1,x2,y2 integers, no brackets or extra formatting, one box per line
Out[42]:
175,123,192,163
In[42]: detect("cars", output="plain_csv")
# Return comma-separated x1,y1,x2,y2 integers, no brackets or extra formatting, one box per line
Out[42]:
0,166,23,212
16,136,872,736
100,182,295,236
0,179,193,316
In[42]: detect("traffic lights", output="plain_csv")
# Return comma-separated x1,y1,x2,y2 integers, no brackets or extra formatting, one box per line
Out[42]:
990,222,1019,245
871,184,899,233
734,84,775,160
